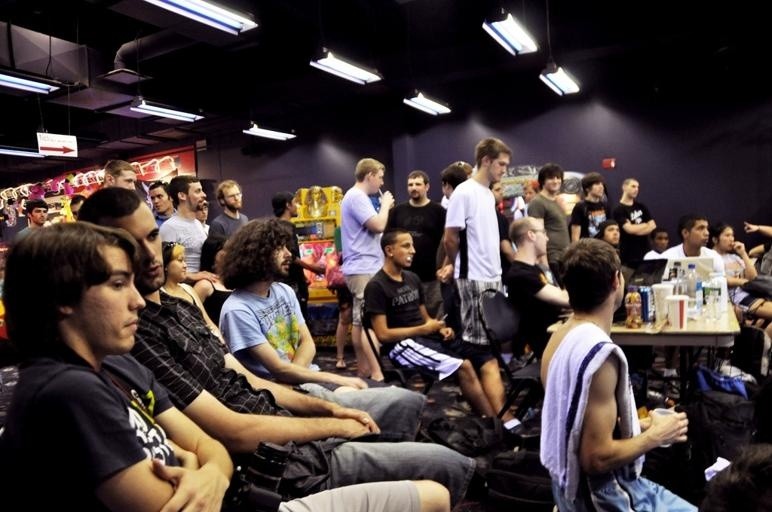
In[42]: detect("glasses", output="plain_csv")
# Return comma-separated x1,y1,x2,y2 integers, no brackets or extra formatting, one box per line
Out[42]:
530,227,547,234
161,241,180,252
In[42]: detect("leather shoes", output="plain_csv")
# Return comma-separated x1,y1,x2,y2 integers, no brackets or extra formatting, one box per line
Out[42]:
511,424,540,439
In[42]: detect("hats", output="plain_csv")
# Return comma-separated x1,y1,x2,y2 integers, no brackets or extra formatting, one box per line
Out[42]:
594,220,618,238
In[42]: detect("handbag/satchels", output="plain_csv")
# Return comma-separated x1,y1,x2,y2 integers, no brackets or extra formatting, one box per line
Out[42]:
685,390,754,460
432,414,514,457
326,251,345,289
695,363,748,397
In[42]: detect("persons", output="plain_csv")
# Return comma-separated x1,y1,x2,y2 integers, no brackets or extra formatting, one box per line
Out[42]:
0,135,771,512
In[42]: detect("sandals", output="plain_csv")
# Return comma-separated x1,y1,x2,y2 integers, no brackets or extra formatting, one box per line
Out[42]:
335,356,345,368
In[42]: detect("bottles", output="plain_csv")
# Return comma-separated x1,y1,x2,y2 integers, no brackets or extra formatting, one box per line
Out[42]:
626,286,641,327
717,272,728,310
685,264,703,318
668,268,679,296
705,273,721,320
673,262,685,291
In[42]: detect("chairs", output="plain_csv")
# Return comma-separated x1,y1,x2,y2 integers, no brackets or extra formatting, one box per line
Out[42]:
358,300,433,398
476,288,544,435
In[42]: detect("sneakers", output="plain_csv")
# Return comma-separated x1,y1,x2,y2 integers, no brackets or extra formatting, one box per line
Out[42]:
664,374,680,400
508,350,534,371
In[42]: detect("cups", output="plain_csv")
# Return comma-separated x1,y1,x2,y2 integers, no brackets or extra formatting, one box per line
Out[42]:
653,409,676,449
653,283,674,320
379,194,395,210
668,295,687,330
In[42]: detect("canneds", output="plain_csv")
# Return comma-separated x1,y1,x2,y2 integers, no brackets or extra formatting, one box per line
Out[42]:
639,286,656,322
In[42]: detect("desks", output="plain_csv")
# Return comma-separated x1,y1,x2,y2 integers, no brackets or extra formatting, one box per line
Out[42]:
546,289,739,462
